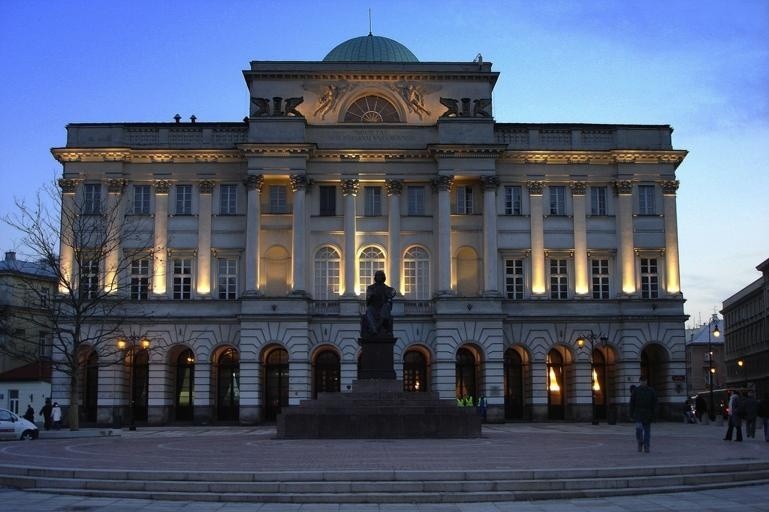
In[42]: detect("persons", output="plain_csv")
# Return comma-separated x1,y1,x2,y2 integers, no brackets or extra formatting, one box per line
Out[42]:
395,84,431,120
366,271,396,337
630,376,656,453
457,394,488,417
722,390,769,442
312,84,337,120
685,394,706,424
25,400,61,431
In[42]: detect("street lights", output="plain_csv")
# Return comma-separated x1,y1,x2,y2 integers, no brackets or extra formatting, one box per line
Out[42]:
708,317,720,420
576,335,609,426
736,359,748,389
117,336,152,433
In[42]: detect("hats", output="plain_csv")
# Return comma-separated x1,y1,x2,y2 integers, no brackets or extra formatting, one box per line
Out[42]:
639,376,647,381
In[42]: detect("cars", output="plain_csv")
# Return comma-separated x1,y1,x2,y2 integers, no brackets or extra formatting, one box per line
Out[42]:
0,408,39,441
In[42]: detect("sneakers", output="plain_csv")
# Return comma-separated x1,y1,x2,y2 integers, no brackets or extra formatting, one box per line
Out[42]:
722,437,743,443
637,443,651,454
